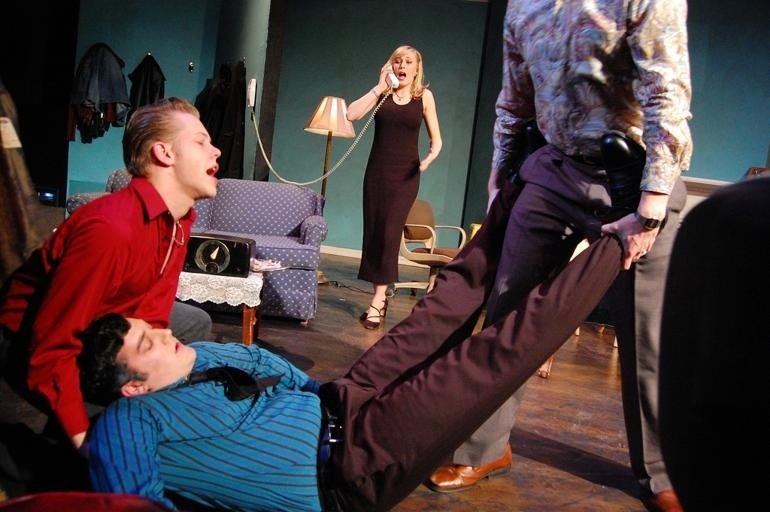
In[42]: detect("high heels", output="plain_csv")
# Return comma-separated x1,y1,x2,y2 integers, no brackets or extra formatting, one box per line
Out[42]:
359,296,390,332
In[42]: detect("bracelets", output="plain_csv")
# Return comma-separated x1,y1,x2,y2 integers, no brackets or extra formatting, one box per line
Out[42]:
427,149,437,159
372,89,379,99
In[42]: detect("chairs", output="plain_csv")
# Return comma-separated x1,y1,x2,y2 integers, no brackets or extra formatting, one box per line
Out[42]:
392,197,467,296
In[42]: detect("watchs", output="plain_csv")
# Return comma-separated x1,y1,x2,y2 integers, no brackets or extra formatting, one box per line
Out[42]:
635,211,665,232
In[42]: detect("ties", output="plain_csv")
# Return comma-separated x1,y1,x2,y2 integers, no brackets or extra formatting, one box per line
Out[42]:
180,366,285,402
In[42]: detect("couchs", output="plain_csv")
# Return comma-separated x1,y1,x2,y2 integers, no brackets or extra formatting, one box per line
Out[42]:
64,167,327,330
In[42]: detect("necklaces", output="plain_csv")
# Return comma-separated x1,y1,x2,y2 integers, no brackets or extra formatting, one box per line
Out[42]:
171,219,185,246
395,91,410,101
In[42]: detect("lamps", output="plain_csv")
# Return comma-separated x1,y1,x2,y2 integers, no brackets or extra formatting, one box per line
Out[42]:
301,95,357,284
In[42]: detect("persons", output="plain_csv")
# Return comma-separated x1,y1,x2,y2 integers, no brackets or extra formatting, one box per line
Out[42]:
421,0,694,512
0,98,223,489
76,123,670,512
657,173,770,512
346,44,442,329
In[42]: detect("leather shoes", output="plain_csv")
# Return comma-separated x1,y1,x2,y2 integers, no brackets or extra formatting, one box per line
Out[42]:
508,121,546,184
598,130,669,232
425,440,513,496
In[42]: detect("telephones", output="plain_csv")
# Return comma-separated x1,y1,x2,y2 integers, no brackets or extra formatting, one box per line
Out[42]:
385,59,400,88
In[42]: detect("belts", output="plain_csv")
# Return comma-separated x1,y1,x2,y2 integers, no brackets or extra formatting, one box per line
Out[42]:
313,380,344,509
